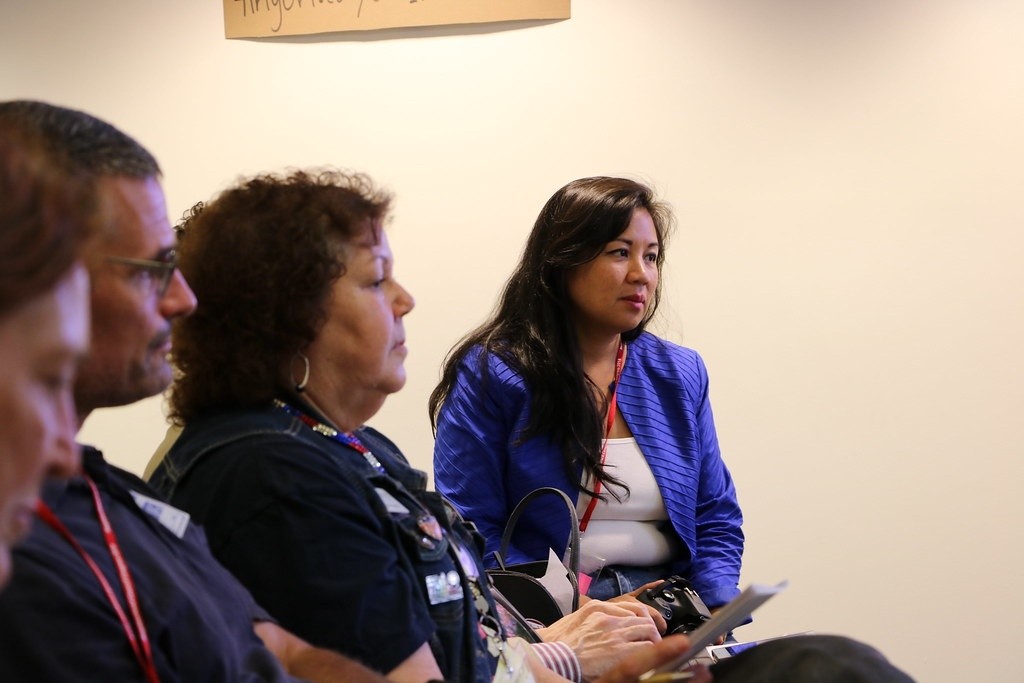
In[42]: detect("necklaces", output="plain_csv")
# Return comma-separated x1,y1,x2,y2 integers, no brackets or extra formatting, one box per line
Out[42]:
34,467,158,683
273,398,386,474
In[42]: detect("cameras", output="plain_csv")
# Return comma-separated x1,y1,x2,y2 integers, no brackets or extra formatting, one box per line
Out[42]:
634,575,713,637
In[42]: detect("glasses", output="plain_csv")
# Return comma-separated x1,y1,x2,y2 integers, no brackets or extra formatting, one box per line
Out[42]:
91,250,182,295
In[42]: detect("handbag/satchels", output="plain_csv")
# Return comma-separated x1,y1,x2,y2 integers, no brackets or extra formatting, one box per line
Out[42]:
471,484,581,626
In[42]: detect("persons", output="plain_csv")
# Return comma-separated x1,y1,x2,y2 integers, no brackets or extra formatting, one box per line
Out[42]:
0,100,921,683
